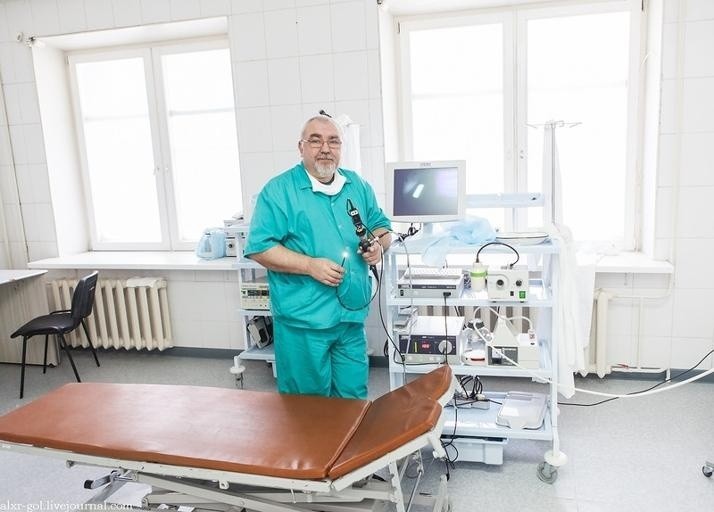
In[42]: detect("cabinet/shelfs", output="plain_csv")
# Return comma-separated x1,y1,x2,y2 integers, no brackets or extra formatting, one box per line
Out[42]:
220,220,277,389
383,244,562,485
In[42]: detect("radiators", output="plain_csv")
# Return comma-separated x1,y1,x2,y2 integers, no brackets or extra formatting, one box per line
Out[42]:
417,286,612,378
42,277,175,353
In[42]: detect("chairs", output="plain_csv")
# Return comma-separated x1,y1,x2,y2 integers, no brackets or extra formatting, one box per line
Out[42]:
9,275,98,399
0,364,457,512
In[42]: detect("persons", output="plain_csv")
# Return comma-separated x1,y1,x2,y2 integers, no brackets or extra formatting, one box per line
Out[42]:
243,117,393,401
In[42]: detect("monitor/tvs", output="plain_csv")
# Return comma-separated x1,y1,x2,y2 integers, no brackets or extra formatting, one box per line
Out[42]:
384,160,467,235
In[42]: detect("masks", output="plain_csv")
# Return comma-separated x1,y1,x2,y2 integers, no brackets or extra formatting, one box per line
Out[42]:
305,169,346,195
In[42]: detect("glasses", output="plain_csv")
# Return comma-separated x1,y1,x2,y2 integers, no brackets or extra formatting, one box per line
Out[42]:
303,139,342,148
